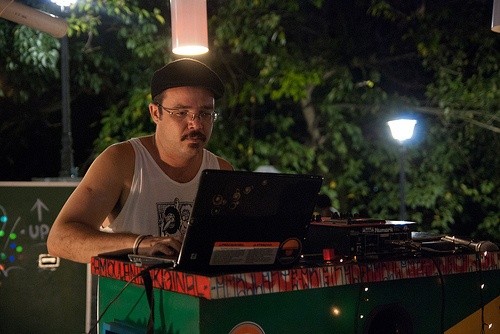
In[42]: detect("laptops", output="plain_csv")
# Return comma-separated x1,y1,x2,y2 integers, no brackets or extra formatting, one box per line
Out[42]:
127,168,324,274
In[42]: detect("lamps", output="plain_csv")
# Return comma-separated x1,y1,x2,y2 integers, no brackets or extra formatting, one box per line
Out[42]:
491,0,500,34
387,114,418,218
169,0,210,55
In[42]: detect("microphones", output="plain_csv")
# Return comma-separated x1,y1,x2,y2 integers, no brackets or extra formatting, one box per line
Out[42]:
442,235,499,253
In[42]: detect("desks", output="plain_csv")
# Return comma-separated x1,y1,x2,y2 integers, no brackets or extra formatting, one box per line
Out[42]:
88,252,500,334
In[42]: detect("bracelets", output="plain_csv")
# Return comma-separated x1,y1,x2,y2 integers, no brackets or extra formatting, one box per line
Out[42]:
133,234,151,255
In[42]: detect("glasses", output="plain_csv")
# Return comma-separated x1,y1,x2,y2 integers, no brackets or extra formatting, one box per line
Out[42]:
157,104,218,122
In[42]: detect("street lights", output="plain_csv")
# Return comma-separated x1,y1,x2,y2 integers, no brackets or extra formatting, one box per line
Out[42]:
386,114,418,221
49,0,81,181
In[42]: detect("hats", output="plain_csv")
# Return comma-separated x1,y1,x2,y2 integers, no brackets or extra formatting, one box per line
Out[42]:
149,57,225,100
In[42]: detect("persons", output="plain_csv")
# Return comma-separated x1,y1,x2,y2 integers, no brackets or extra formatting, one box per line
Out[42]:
46,58,233,263
